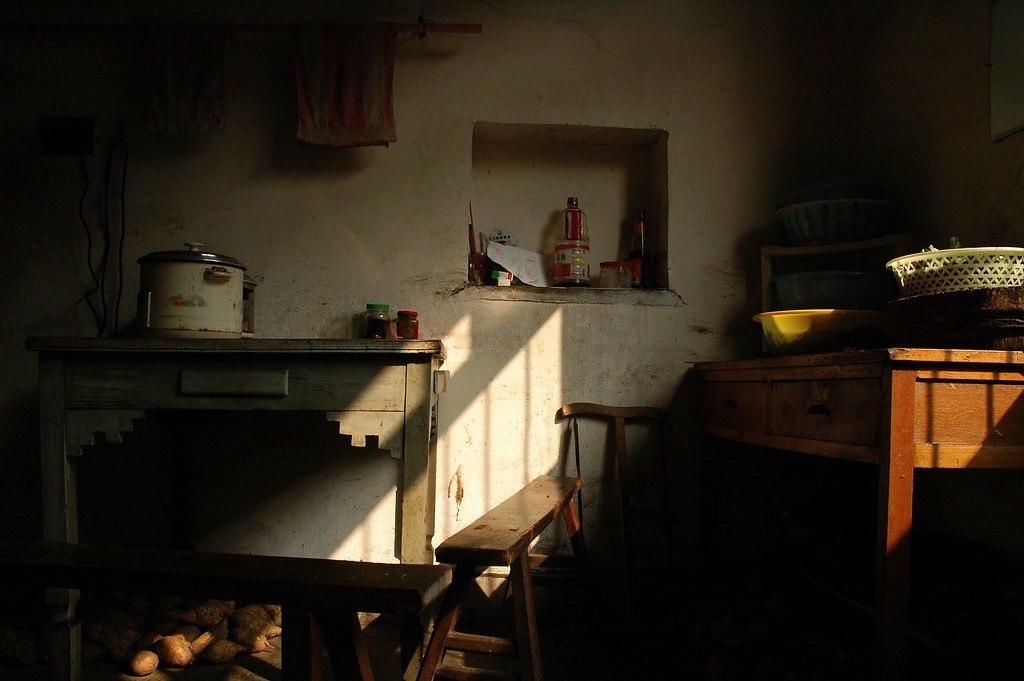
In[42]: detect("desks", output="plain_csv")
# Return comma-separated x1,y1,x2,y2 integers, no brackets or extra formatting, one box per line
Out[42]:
692,344,1024,681
25,335,444,681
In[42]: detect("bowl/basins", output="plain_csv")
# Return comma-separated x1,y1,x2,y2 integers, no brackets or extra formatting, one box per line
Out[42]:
754,309,869,357
776,199,899,245
772,272,873,309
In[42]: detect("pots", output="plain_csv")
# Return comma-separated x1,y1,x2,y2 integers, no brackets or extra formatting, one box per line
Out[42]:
242,271,264,340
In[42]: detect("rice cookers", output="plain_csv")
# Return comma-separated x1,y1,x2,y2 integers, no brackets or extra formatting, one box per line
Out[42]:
136,240,248,340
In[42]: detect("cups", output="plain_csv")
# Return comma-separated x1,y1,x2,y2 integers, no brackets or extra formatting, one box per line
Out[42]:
599,260,630,289
469,252,486,285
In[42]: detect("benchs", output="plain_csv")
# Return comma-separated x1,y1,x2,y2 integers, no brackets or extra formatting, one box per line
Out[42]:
0,547,455,681
415,475,603,681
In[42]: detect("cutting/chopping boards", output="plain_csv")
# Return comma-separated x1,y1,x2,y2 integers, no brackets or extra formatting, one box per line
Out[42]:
883,287,1024,354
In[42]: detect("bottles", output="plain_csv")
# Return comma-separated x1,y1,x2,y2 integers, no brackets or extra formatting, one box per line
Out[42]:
553,198,590,287
629,219,649,287
